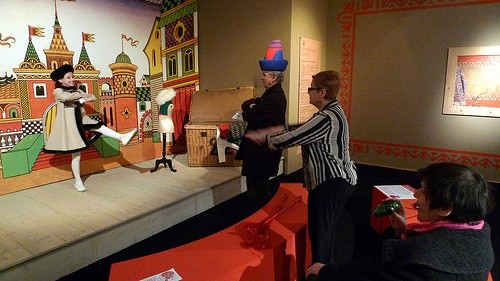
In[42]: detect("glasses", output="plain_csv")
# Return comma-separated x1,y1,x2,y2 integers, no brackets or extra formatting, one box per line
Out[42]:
308,87,325,91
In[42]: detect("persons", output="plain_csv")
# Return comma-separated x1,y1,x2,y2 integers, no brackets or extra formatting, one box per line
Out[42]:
245,71,358,266
235,66,286,211
44,63,138,192
155,88,176,133
304,162,495,281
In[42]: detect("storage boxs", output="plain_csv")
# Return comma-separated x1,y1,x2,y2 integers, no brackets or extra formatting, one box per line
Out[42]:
183,87,258,167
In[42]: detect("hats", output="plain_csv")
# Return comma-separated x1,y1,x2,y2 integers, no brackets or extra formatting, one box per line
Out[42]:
259,39,288,71
51,63,74,80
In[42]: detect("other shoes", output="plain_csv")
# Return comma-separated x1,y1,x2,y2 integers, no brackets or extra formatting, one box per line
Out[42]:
122,129,138,147
74,181,87,191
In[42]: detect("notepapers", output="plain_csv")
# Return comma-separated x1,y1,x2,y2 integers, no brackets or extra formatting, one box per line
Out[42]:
140,266,186,281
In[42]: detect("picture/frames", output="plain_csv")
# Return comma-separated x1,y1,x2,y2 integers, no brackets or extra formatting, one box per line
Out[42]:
443,47,500,117
